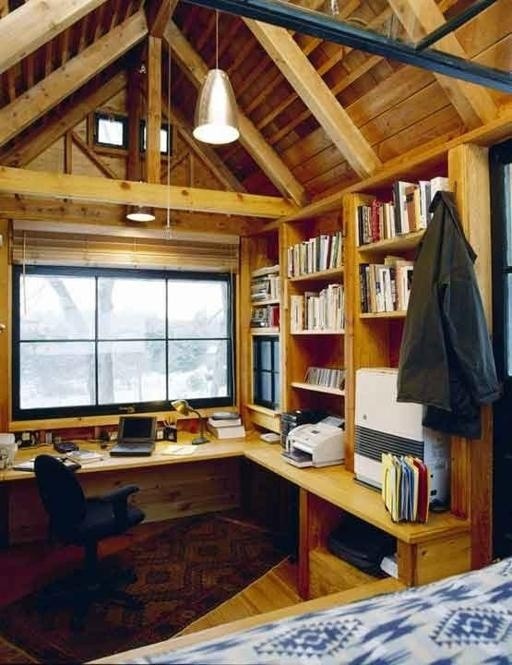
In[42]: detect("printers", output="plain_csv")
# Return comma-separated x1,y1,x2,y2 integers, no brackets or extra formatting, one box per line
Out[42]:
280,423,345,469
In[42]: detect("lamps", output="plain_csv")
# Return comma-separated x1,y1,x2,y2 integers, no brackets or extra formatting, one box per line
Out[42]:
126,64,156,222
171,400,210,444
192,10,241,145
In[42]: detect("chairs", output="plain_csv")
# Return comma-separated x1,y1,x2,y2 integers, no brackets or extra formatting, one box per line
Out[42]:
27,454,145,630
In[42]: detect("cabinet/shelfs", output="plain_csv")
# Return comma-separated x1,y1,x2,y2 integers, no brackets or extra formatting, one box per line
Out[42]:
283,189,391,471
352,143,493,521
240,217,317,436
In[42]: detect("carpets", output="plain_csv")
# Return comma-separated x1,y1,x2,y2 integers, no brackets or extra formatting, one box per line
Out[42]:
0,506,299,665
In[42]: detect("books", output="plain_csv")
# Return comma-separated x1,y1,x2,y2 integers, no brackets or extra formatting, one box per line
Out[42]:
357,256,417,312
12,456,64,471
68,450,102,465
288,232,343,275
290,283,347,331
248,273,279,328
208,413,246,440
305,365,346,389
355,174,449,248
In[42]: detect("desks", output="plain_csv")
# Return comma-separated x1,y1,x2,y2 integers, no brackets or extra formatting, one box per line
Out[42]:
0,430,472,601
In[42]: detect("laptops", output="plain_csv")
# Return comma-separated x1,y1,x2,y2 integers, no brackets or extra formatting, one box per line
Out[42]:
109,416,157,457
